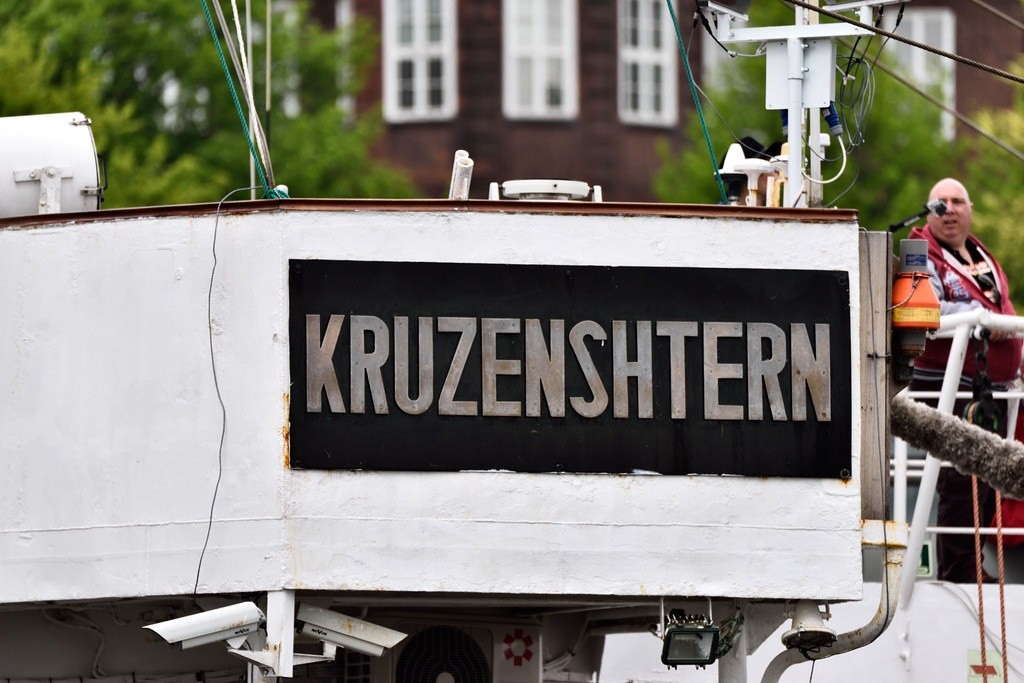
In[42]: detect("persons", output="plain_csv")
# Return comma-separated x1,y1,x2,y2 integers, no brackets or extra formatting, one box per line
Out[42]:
714,135,789,207
910,177,1024,583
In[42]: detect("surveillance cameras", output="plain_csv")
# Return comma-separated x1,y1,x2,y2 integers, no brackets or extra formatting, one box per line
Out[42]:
142,601,259,651
294,601,408,658
